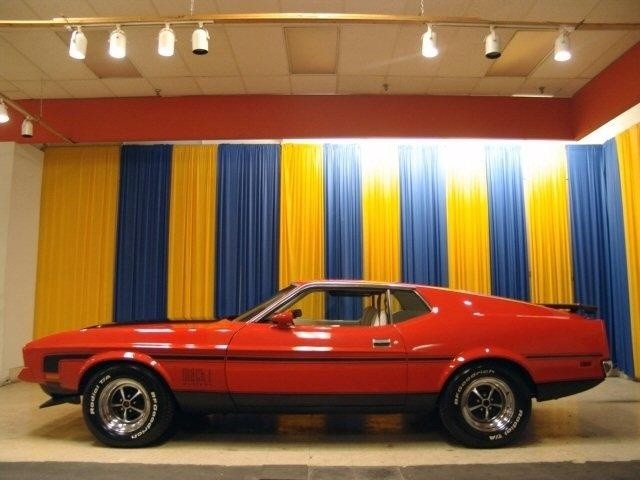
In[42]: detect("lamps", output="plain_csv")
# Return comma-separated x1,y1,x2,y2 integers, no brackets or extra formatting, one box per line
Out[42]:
65,24,88,62
417,28,441,62
190,23,209,54
19,115,37,142
553,29,575,64
153,24,177,57
1,100,11,127
103,24,129,59
484,27,505,60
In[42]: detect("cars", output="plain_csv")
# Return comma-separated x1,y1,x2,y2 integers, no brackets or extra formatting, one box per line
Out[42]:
18,278,614,448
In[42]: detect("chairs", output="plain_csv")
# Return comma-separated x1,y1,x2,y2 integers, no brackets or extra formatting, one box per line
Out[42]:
340,306,387,327
